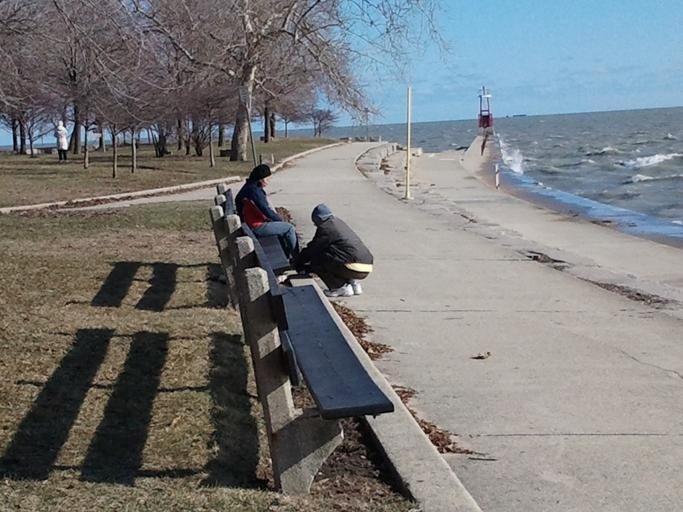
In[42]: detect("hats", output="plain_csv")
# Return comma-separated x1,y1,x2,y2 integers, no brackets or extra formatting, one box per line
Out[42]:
248,164,271,181
311,203,335,221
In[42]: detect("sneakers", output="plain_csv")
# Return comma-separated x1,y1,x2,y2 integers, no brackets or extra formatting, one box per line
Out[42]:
323,284,354,298
348,280,362,295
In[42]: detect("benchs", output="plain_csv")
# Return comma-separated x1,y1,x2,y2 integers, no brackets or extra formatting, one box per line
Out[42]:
210,181,395,496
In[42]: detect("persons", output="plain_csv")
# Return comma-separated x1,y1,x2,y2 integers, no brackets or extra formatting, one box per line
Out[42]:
52,120,69,163
234,164,308,275
288,202,373,298
